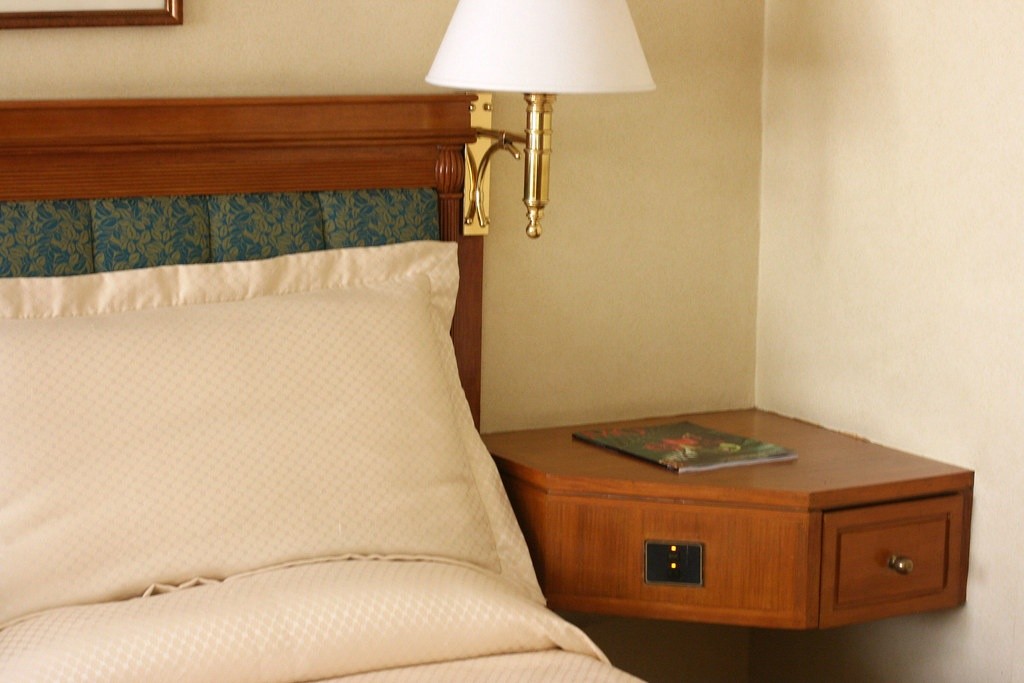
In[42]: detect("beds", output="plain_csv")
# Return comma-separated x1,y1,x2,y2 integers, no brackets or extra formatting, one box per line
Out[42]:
0,91,648,682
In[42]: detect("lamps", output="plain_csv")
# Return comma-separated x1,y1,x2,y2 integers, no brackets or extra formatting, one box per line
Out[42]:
425,2,655,238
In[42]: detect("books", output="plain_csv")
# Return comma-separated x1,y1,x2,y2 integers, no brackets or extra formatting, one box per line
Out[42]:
572,420,797,476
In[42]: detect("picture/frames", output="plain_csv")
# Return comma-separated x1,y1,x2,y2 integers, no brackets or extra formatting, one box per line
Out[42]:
0,0,184,31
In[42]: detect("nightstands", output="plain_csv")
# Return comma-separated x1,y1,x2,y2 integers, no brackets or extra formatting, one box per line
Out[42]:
479,408,975,631
